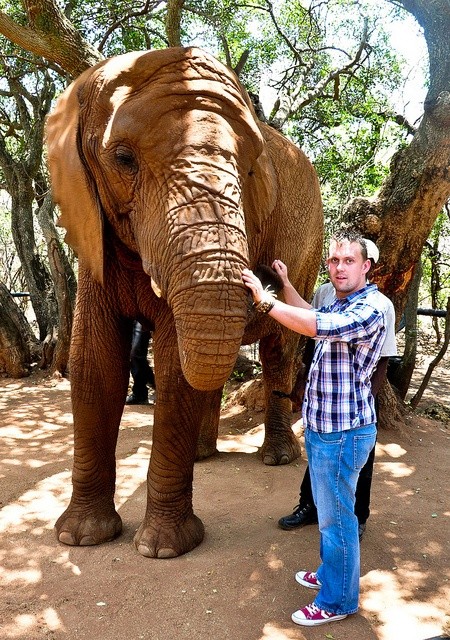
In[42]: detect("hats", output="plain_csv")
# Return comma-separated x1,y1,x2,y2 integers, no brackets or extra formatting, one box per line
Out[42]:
363,238,380,264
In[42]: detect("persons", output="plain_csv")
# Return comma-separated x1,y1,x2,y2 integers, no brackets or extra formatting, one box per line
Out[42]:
123,318,158,406
276,235,399,543
240,232,390,628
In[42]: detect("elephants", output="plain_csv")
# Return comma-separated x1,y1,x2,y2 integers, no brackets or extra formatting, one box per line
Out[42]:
41,43,325,562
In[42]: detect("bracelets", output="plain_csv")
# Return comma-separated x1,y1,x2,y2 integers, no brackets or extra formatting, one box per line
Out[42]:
251,284,277,315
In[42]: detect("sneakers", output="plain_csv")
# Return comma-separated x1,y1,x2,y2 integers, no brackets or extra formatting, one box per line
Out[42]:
359,523,366,542
278,504,318,530
125,395,148,405
291,601,348,627
295,571,322,589
